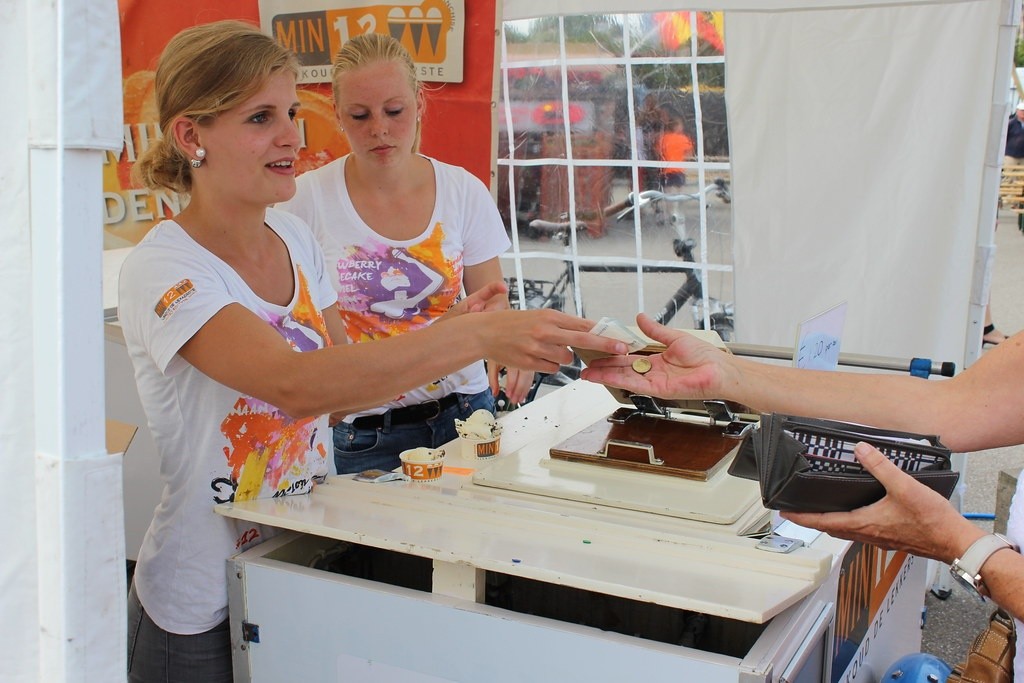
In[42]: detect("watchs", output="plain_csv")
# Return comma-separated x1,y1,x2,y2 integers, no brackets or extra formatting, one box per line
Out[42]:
949,531,1021,602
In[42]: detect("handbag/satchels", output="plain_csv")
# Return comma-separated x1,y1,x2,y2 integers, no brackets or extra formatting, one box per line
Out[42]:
946,610,1017,683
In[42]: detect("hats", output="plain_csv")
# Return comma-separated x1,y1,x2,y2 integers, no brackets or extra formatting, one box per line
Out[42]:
1016,103,1024,110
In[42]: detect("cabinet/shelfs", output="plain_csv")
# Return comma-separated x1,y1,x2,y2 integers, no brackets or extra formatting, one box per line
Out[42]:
217,381,928,683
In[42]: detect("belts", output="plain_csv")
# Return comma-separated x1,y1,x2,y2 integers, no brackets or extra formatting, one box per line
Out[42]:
353,393,468,430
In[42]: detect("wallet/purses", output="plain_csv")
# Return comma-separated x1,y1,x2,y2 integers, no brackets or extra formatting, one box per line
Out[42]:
726,413,961,512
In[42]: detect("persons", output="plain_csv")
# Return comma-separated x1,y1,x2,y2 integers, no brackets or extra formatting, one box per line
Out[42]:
1005,103,1024,160
274,31,535,478
981,287,1010,349
580,312,1024,683
117,19,628,683
637,89,695,226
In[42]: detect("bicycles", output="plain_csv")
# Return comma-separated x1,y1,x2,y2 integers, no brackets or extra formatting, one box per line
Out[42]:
503,177,734,342
